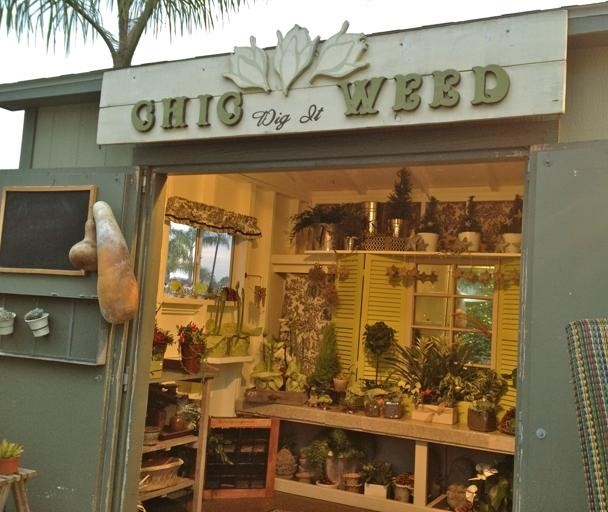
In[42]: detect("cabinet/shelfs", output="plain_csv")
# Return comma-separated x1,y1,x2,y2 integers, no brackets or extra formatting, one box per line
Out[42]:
137,370,217,512
203,418,280,497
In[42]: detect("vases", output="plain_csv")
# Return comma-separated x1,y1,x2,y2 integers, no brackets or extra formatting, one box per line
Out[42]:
149,353,164,379
181,342,205,375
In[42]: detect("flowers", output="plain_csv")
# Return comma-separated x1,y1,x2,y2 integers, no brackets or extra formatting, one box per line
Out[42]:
177,321,210,344
152,319,176,354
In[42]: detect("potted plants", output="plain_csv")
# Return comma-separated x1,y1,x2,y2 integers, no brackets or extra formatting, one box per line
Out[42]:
0,307,16,335
384,336,467,425
393,472,414,503
1,439,23,474
206,286,227,359
501,194,523,253
276,428,364,494
228,282,249,356
245,323,341,409
285,203,368,250
467,398,497,432
25,308,50,338
363,458,393,499
333,320,403,419
386,168,413,237
417,196,443,252
458,195,482,252
